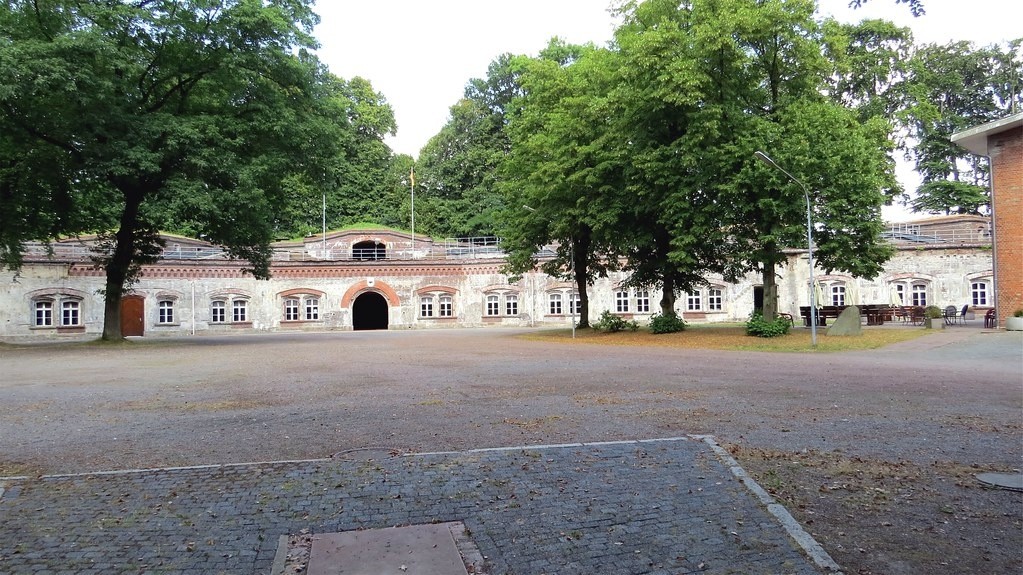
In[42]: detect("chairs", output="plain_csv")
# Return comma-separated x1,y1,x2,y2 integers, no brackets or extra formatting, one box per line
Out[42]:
910,304,968,326
984,308,996,328
777,312,795,328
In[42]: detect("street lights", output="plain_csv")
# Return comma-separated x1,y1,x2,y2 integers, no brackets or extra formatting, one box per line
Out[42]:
408,166,417,261
753,150,818,346
522,204,576,339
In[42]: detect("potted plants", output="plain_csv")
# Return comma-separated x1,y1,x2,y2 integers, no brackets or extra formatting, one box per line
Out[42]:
1004,308,1023,331
924,306,943,330
